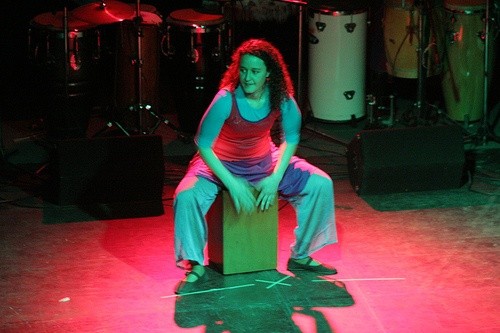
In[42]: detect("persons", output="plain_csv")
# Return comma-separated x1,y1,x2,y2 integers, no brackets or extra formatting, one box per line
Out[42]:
174,39,339,293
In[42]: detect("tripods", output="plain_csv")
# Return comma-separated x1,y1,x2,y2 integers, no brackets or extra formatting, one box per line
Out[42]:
390,0,481,146
93,0,191,145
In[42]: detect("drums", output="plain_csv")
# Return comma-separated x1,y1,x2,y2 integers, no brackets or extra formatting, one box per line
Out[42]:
307,0,368,123
382,0,435,79
165,8,235,88
27,10,114,97
441,0,495,124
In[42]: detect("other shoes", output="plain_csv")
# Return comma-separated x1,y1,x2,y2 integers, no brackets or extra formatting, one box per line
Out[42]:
174,268,208,295
287,256,337,274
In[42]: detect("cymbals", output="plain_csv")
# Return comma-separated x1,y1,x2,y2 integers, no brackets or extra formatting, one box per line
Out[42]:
70,0,139,26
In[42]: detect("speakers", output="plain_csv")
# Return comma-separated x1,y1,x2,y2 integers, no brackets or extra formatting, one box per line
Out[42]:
346,122,464,196
32,134,164,206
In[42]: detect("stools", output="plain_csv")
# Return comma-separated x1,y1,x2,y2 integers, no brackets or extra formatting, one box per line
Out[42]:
208,191,277,274
